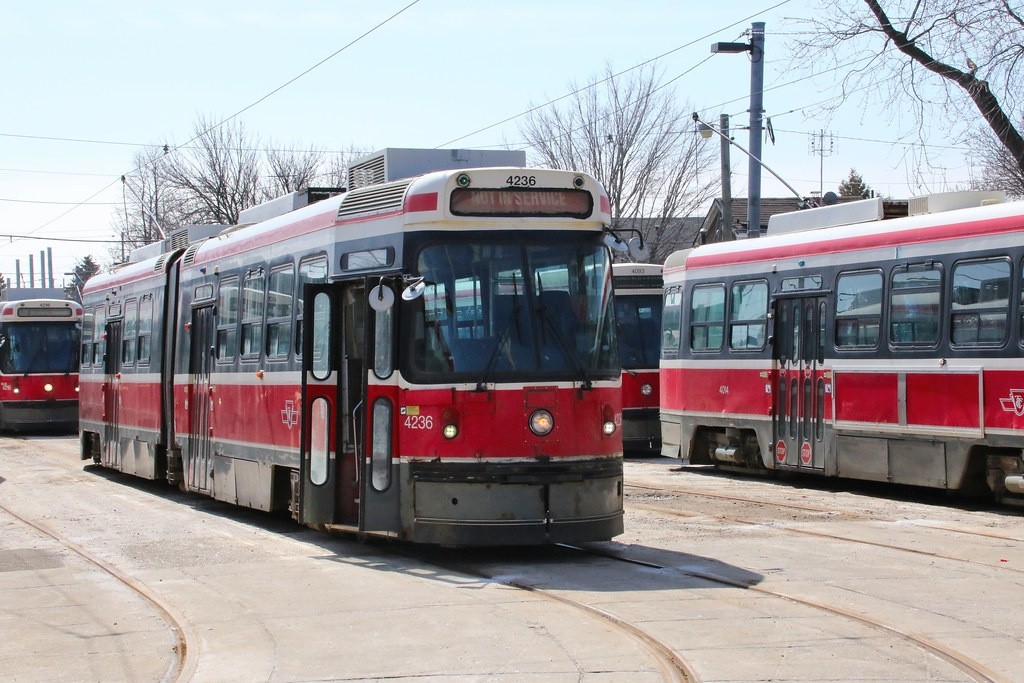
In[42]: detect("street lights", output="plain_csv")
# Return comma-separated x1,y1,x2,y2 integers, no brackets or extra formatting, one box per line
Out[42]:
709,21,767,237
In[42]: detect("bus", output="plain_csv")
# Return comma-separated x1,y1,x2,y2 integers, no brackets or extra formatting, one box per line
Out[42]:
0,299,84,433
78,148,648,556
424,262,666,455
656,191,1024,511
